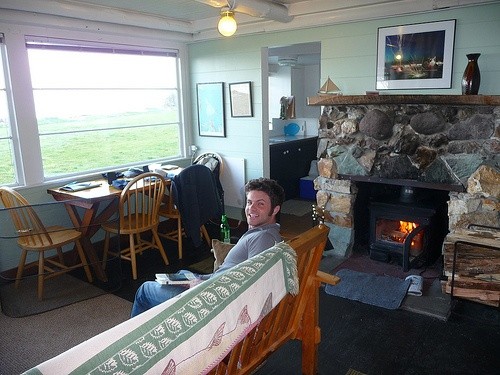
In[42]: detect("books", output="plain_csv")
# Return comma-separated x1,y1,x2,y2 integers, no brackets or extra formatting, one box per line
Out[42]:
154,274,199,285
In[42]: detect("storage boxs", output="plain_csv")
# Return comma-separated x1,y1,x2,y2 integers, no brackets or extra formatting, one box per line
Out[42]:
299,176,316,201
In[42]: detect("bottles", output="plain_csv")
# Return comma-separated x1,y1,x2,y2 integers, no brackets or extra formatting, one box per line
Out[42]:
219,215,230,243
462,53,481,95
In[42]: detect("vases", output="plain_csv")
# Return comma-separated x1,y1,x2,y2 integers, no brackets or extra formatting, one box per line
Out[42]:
461,52,481,94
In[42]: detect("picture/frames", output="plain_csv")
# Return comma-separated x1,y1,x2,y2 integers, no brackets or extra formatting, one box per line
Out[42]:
229,81,252,117
375,19,456,90
197,82,225,136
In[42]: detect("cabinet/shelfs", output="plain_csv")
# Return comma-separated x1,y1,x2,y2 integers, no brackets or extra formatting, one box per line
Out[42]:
268,144,296,200
294,140,318,196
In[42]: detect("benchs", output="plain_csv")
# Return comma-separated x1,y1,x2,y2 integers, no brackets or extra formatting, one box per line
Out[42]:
22,225,341,375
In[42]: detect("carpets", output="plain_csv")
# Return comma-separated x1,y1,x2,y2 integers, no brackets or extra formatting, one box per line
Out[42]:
324,269,411,312
54,213,253,304
0,267,109,318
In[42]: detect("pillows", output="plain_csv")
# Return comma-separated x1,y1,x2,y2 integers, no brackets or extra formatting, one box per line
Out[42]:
211,239,235,272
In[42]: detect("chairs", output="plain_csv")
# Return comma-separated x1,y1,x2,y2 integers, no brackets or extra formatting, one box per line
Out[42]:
194,153,224,177
101,172,169,280
151,166,220,260
0,187,92,300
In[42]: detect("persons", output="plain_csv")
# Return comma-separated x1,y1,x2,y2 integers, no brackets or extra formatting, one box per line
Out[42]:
130,177,285,319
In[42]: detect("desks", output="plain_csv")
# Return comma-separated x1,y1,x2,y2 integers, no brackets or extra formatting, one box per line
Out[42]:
46,166,187,282
